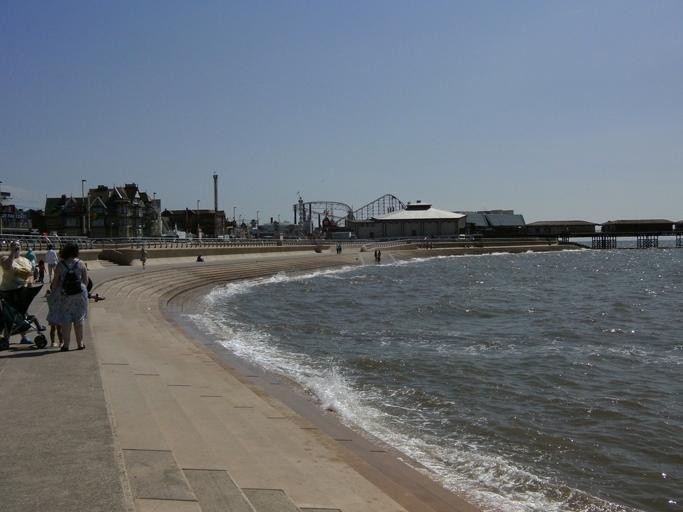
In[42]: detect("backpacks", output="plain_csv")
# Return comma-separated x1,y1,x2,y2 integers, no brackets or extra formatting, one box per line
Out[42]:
60,258,81,296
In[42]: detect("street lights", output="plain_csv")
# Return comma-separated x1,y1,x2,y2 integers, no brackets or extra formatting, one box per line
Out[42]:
81,179,280,239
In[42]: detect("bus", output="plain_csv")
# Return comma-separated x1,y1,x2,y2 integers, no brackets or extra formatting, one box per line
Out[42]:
331,232,352,239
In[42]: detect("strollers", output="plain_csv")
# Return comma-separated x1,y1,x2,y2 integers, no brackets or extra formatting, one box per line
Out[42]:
0,284,48,351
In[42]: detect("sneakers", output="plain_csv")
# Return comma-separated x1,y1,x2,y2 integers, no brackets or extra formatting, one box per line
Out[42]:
21,337,34,344
51,343,85,351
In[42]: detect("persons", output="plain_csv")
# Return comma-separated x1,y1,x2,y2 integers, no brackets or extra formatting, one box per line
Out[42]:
138,247,150,269
336,239,342,255
196,254,204,262
374,250,377,262
378,250,381,263
0,239,106,352
